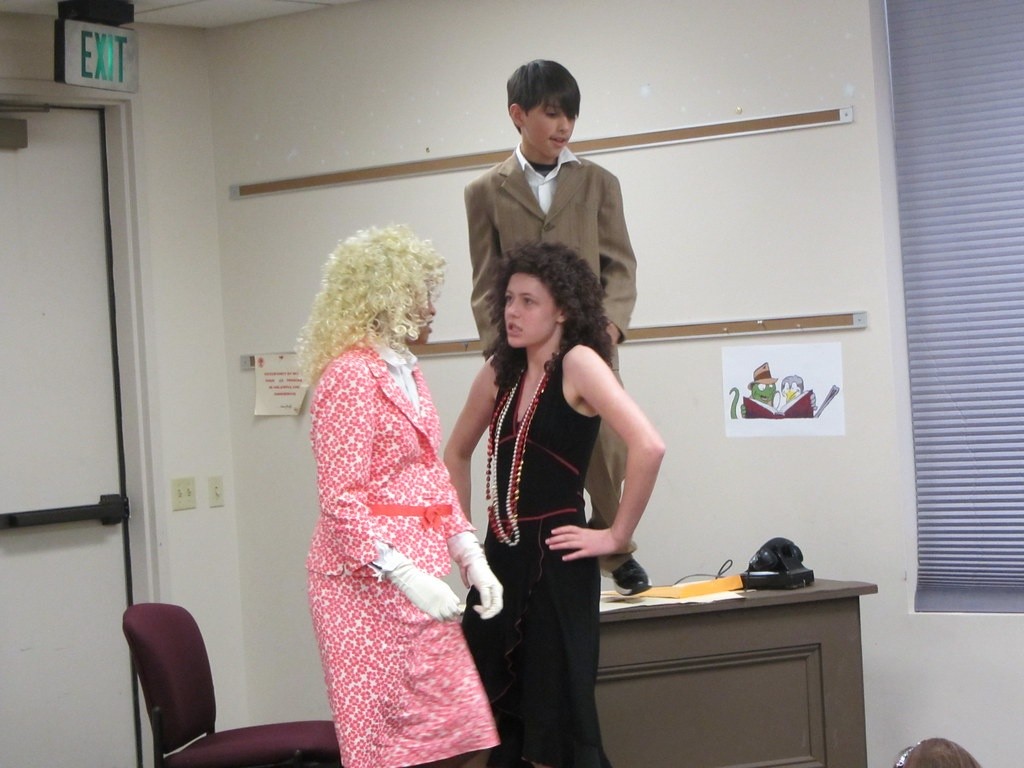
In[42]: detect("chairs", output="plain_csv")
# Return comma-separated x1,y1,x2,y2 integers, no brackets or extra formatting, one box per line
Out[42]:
123,603,342,768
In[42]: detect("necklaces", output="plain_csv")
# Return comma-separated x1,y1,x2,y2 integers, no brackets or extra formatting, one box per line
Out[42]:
486,360,549,547
528,161,557,172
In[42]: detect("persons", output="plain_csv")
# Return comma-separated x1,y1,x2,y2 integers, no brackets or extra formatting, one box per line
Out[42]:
440,240,665,768
458,57,655,600
300,226,510,767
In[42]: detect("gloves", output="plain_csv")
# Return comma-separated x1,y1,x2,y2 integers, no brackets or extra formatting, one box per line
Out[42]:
370,549,465,623
448,532,504,620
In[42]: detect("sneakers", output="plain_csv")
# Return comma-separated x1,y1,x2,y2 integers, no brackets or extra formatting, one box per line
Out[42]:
603,560,653,596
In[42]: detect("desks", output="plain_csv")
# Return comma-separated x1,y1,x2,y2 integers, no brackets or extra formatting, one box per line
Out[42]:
598,579,878,767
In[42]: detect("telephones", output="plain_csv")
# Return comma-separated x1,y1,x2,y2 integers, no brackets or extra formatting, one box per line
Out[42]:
740,538,814,591
